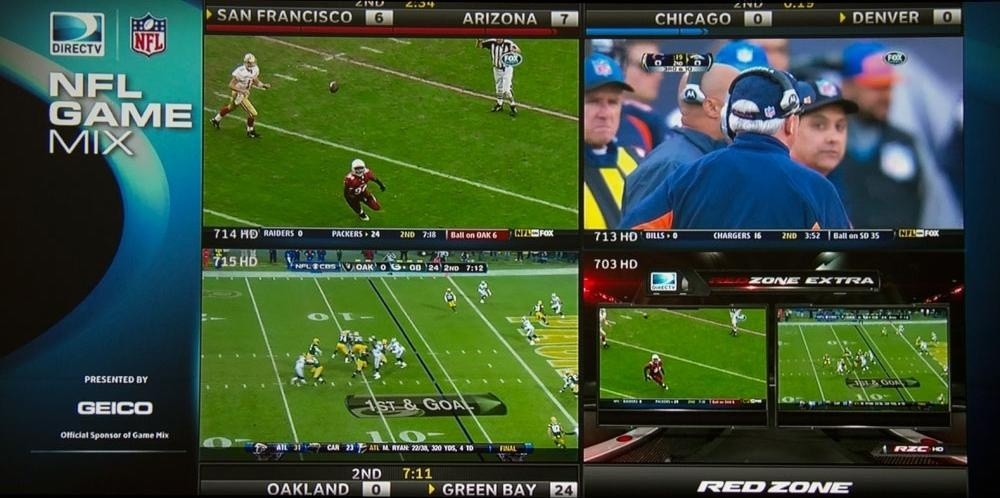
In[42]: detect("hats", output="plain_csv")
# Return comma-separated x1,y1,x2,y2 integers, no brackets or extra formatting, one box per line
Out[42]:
585,53,633,95
714,41,900,120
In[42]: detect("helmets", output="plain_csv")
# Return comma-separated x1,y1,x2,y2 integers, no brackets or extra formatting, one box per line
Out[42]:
651,355,658,362
244,54,257,70
352,159,365,176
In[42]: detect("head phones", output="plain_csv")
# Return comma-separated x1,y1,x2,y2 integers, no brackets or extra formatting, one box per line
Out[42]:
681,72,705,104
721,66,798,142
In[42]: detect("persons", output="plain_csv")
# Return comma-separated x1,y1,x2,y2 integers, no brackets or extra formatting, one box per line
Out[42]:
476,38,522,116
444,288,457,311
520,292,564,345
823,347,876,371
290,330,407,387
268,249,277,264
547,417,576,448
881,324,937,352
203,248,210,267
644,354,669,390
583,39,924,229
209,52,271,137
729,308,747,337
560,371,579,398
477,280,491,303
285,249,550,264
599,307,616,349
343,159,385,221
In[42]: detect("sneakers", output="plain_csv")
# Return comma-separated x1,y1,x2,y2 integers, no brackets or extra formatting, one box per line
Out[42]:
247,130,260,138
359,211,369,221
491,103,518,116
209,119,221,129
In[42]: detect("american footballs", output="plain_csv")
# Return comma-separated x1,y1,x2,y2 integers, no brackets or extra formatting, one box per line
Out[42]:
329,81,339,93
